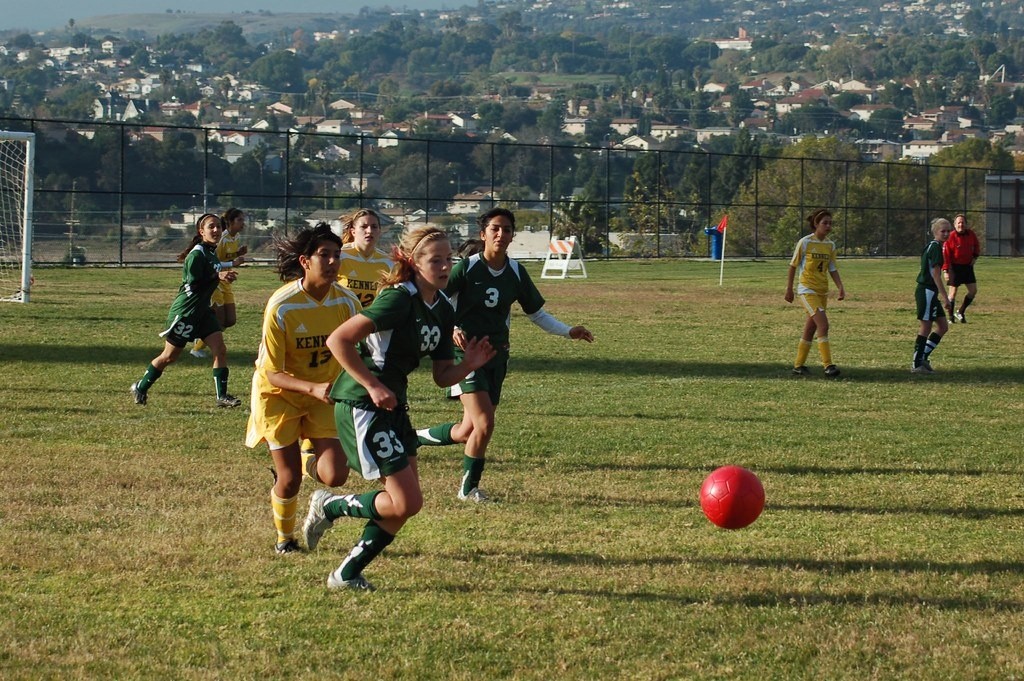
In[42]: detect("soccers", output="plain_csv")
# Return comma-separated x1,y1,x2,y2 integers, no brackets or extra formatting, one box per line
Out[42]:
699,465,765,530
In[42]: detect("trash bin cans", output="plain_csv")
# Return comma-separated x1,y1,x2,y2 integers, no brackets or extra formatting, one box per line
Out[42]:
704,226,724,259
70,254,86,266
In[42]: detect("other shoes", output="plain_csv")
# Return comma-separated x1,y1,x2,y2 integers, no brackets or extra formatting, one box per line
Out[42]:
912,365,934,374
948,320,955,324
956,310,966,323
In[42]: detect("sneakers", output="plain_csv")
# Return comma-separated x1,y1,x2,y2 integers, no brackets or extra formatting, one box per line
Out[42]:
218,394,241,407
824,365,840,377
275,538,303,554
327,571,377,592
457,487,484,500
305,490,334,550
793,365,811,376
131,381,146,405
190,348,206,358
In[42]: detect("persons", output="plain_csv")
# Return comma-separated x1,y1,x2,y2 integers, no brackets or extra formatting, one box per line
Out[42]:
784,209,845,374
913,217,952,373
129,207,247,407
942,213,980,324
244,208,595,590
